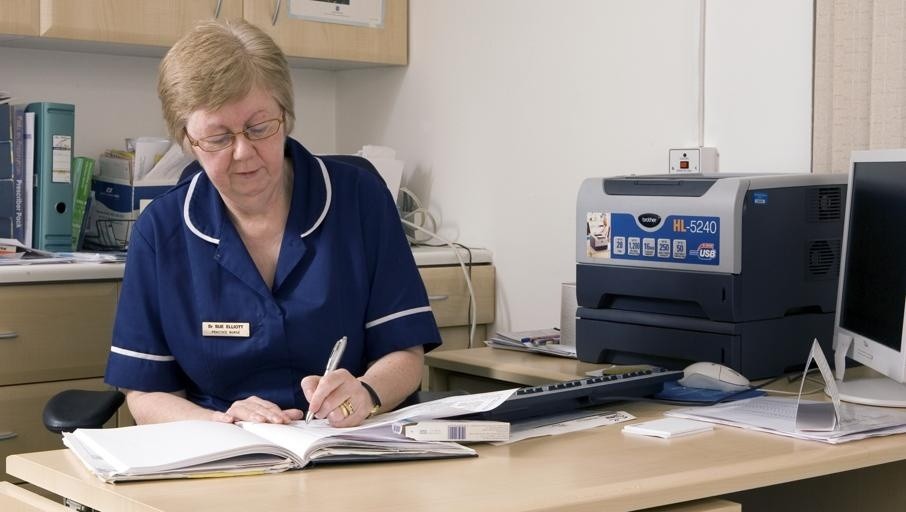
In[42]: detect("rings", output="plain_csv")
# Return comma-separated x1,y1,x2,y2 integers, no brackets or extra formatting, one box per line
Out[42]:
340,399,353,417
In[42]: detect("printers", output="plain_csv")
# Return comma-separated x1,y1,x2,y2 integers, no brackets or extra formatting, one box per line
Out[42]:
576,172,852,381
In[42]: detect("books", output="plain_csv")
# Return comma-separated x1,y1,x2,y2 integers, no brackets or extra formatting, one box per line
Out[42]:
66,415,481,487
10,107,26,246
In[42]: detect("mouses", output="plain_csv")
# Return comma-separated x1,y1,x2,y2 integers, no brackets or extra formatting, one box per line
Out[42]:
681,361,751,394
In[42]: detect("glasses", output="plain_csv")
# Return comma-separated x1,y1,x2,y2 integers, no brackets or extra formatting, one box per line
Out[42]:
182,102,285,153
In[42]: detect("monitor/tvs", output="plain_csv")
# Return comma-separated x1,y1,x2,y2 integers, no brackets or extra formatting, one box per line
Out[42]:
824,148,906,409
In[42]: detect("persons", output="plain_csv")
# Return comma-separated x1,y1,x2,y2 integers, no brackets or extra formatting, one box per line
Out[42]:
108,15,442,427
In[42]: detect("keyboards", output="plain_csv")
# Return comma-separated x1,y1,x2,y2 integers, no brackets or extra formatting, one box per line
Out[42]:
439,368,683,422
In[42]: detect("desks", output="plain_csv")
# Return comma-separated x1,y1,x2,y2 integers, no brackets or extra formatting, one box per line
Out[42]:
9,340,906,512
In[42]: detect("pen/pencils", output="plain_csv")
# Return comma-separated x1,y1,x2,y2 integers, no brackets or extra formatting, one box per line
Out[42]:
304,336,348,425
520,334,560,346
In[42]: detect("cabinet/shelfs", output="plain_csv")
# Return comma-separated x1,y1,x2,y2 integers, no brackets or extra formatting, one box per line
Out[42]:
2,0,407,62
418,263,497,377
0,278,129,477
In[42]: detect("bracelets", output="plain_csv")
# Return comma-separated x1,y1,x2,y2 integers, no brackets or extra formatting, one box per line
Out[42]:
356,375,385,419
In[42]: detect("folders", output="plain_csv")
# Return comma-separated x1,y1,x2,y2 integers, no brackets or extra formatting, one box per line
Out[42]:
25,101,79,254
